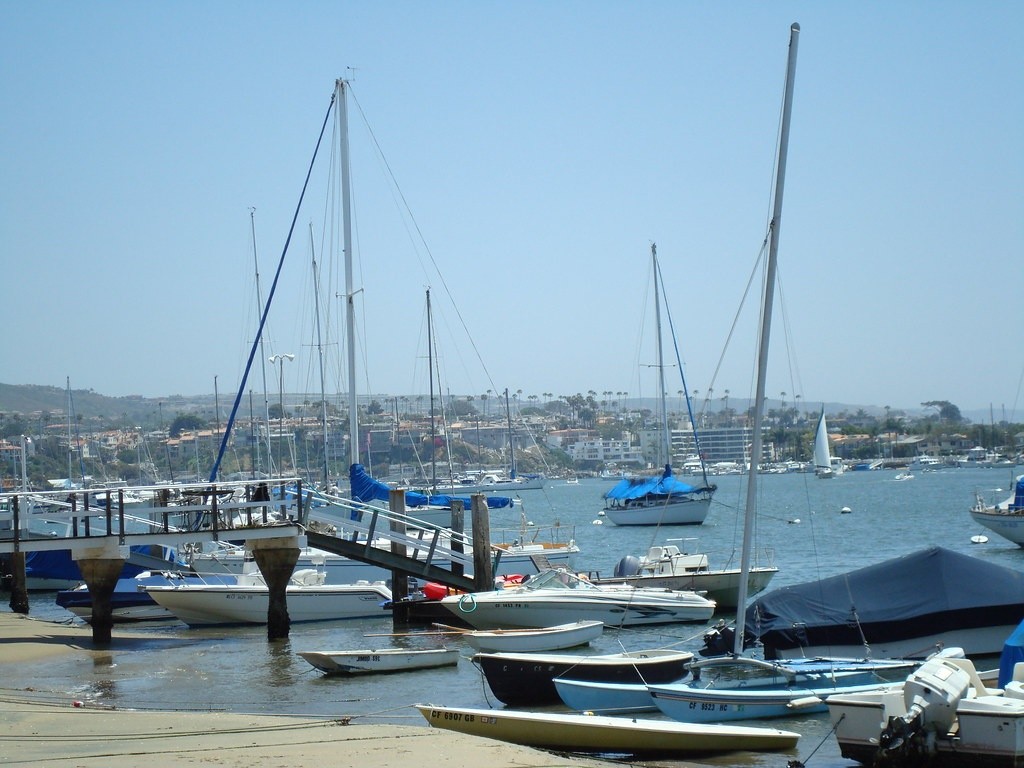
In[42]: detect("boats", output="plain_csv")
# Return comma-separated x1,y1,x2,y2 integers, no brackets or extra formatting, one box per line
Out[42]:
955,403,1015,468
416,703,802,751
295,648,461,676
441,569,717,631
553,22,927,725
379,538,780,628
969,474,1024,546
698,546,1024,660
895,473,914,481
462,620,604,653
472,649,694,706
0,63,580,629
908,448,942,469
825,619,1024,768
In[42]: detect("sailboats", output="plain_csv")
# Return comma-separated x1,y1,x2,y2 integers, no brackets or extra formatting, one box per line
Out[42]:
568,477,578,485
813,402,833,479
603,242,717,526
